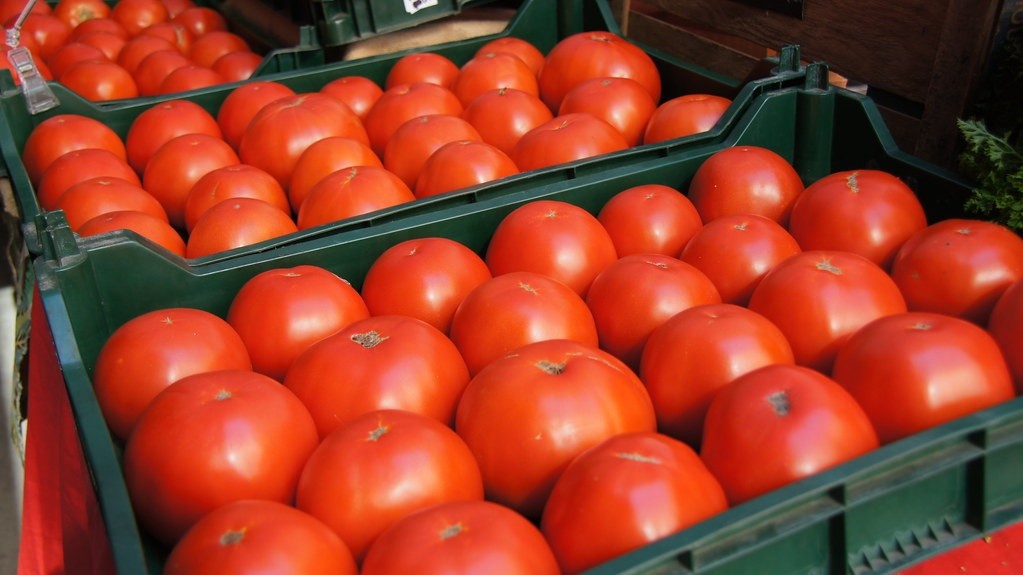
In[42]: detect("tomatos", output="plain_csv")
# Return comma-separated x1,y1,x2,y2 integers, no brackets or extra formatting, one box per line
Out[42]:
90,141,1023,575
0,0,266,101
23,30,735,259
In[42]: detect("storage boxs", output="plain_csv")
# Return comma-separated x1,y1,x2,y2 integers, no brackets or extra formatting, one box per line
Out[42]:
0,0,1023,575
